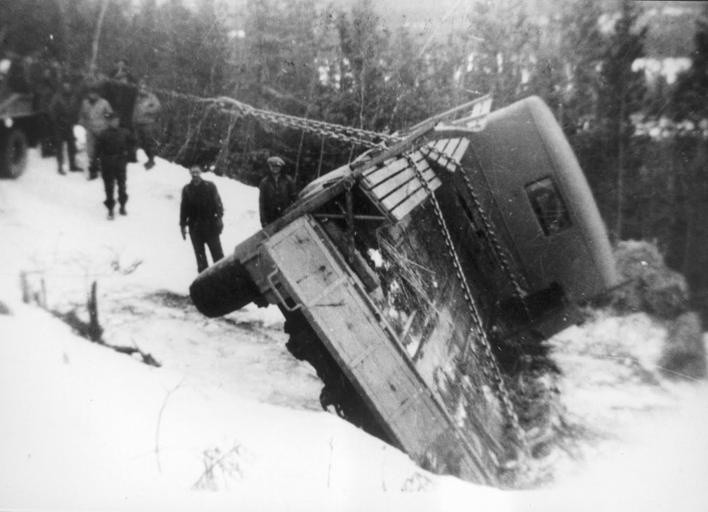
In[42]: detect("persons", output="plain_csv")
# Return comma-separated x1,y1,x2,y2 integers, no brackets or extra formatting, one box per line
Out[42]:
179,165,223,273
89,113,136,220
258,157,297,227
0,38,163,180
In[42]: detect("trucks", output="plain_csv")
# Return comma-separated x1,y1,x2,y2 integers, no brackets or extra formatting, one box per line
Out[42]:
0,56,44,177
190,91,622,489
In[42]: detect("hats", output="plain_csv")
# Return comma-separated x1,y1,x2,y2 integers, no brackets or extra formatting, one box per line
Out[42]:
267,156,286,168
114,56,130,66
104,112,120,119
